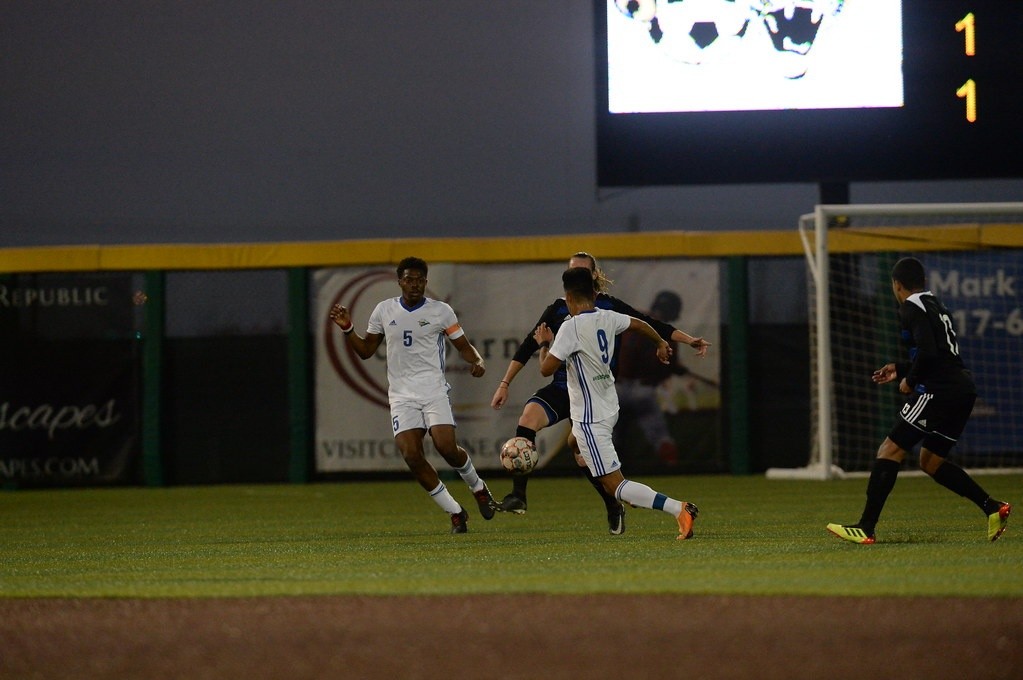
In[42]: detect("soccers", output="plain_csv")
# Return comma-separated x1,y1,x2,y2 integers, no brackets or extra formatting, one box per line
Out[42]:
499,437,540,476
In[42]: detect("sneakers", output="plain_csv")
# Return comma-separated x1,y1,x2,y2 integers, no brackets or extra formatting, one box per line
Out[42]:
488,494,527,515
826,523,877,544
469,479,496,520
451,506,469,533
676,502,698,540
608,504,625,535
987,501,1012,544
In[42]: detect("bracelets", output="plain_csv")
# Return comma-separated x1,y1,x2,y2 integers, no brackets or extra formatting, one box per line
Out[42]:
340,322,354,334
500,381,509,386
539,341,550,349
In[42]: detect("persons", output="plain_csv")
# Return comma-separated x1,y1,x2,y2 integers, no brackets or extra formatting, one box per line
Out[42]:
827,255,1012,545
490,252,713,541
329,258,496,534
